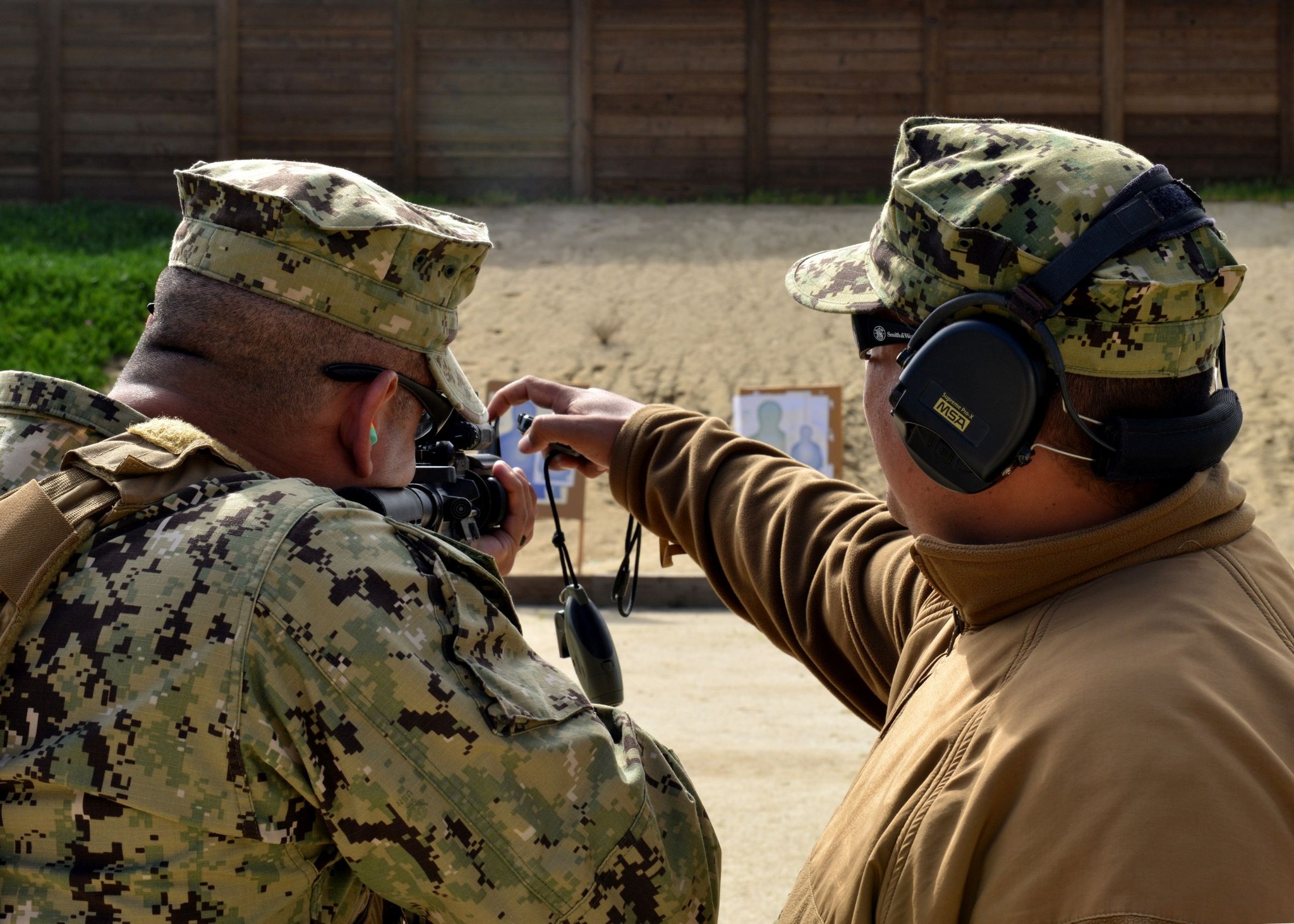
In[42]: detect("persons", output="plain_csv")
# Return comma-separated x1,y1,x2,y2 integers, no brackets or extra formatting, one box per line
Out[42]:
488,116,1294,924
0,159,720,924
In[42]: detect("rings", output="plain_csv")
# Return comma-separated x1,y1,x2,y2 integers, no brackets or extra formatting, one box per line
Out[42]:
520,535,527,546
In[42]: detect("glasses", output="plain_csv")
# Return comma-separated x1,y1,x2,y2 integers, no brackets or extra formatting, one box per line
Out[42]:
324,363,455,437
853,311,914,361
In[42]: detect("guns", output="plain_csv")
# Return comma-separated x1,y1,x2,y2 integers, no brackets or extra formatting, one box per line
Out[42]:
335,419,508,545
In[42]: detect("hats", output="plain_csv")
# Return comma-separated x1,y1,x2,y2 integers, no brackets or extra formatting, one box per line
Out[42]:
783,116,1246,379
168,158,491,425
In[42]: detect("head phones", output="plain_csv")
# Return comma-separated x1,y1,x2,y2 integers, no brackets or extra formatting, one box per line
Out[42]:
888,165,1243,494
